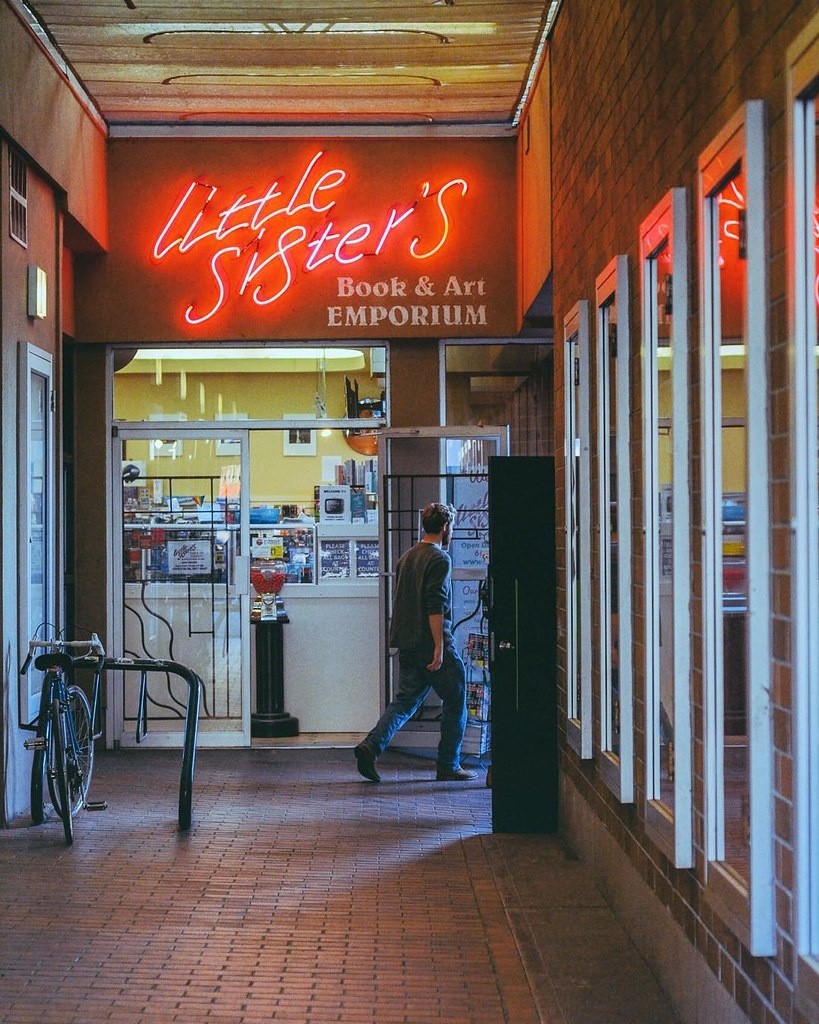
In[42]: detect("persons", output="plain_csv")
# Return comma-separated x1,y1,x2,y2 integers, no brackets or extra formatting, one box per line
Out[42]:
352,500,479,786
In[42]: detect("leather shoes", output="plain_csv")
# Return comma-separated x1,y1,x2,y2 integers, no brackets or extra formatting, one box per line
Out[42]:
354,744,382,783
435,767,480,782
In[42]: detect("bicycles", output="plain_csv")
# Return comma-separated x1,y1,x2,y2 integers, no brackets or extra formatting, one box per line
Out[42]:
20,633,110,847
